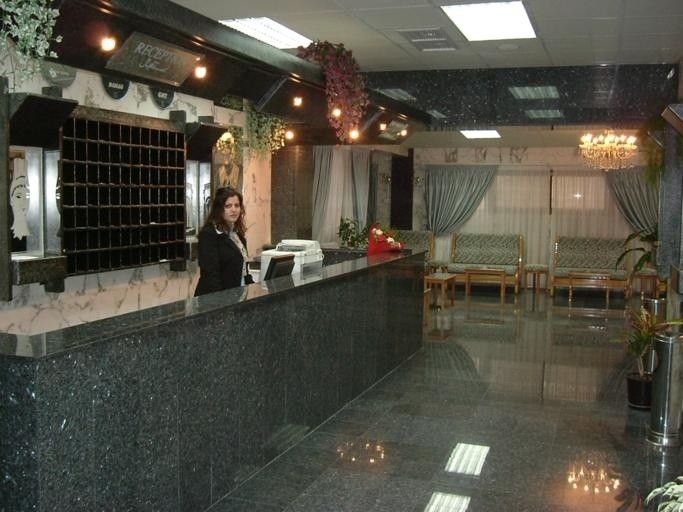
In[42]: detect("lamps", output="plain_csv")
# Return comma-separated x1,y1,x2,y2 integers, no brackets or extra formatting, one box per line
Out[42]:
578,129,638,174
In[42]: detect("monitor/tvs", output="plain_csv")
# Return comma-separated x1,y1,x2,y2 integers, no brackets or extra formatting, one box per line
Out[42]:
264,254,294,281
266,274,295,294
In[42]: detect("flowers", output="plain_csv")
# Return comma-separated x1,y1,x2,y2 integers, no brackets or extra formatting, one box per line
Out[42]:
366,222,406,258
296,36,373,145
0,0,63,101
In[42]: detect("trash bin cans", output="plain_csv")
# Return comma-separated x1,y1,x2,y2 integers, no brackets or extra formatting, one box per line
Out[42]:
645,321,683,445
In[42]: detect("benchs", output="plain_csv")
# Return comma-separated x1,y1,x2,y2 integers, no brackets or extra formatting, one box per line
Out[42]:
398,229,633,303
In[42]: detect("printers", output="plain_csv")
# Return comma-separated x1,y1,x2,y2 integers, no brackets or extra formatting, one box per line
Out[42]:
261,239,324,280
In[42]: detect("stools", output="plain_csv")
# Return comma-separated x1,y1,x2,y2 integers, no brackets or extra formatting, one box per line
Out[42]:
424,272,458,310
633,268,658,301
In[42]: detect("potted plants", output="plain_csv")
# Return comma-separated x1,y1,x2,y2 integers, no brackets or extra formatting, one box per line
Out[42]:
606,308,683,410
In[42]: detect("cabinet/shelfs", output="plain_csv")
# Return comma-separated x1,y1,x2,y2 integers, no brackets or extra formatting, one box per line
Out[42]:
44,105,189,283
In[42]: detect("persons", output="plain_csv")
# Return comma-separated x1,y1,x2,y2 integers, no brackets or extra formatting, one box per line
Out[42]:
193,187,252,297
